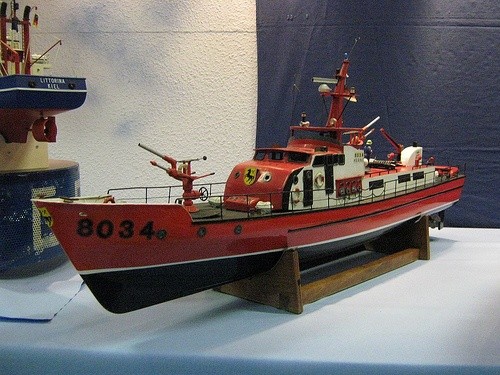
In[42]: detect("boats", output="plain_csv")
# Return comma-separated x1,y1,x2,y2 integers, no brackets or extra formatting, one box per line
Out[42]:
28,34,467,314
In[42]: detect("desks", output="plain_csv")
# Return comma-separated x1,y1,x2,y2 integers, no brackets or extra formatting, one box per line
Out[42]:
0,228,500,375
